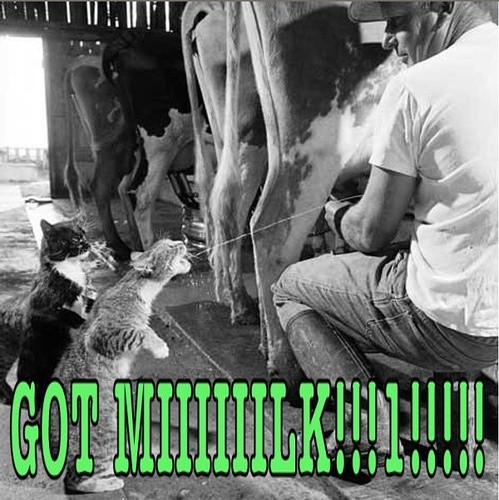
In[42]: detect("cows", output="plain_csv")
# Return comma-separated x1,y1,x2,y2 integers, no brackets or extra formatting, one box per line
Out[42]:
62,55,139,263
208,0,498,410
101,26,219,250
180,1,270,328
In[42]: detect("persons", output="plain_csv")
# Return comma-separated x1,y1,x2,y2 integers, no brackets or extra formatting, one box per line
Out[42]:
271,1,497,471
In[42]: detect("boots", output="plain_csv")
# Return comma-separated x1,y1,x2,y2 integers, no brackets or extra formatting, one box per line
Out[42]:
284,308,406,470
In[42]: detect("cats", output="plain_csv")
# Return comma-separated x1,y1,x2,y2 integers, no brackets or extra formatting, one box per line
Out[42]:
16,213,91,409
1,235,109,406
38,237,192,493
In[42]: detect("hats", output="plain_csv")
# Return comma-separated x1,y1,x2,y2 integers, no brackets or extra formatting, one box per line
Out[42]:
348,1,388,25
